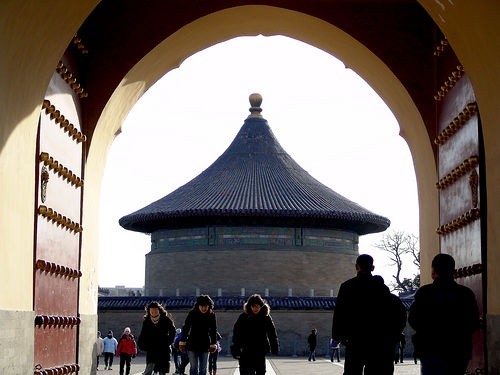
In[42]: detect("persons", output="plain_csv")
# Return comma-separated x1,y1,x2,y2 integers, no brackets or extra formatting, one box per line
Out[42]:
331,254,407,375
103,330,119,370
230,294,280,375
116,327,137,375
408,253,479,375
138,301,176,375
395,332,406,364
179,295,218,375
307,328,318,361
209,331,222,375
96,331,104,371
171,328,190,375
330,341,341,362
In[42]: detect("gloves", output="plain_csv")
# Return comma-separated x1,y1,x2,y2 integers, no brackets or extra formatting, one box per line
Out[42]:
208,344,217,352
132,354,135,358
179,341,186,352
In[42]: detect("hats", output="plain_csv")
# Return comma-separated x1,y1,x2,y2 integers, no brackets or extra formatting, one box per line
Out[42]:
124,327,131,334
